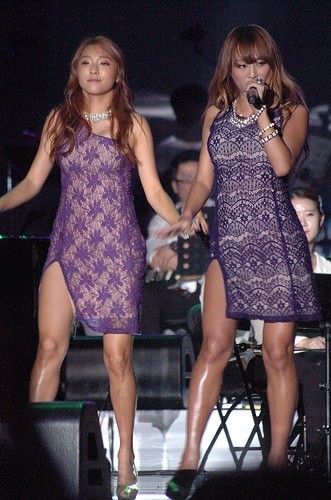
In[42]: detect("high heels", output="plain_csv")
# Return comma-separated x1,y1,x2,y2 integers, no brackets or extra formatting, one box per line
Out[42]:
117,459,139,500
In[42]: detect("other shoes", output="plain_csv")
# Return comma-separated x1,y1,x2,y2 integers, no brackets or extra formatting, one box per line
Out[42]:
165,469,198,500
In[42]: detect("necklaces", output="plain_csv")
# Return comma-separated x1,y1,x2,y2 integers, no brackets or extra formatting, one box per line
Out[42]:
233,98,256,125
83,110,112,121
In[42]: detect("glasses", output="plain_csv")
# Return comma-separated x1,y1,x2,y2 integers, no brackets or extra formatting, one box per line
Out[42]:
176,177,196,184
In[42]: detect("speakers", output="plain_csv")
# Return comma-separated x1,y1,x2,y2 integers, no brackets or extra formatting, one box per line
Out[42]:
184,470,331,500
57,334,182,410
0,402,113,500
298,349,331,467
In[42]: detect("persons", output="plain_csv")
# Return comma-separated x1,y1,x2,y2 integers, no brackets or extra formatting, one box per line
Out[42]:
152,24,320,500
250,189,331,456
144,157,214,309
0,36,208,500
191,468,331,500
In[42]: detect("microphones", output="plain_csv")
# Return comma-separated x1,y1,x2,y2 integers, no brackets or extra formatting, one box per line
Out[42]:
245,87,258,105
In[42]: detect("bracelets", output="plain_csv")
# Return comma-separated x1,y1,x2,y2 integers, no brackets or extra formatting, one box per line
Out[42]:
254,104,267,121
177,216,190,239
259,123,275,136
260,129,280,144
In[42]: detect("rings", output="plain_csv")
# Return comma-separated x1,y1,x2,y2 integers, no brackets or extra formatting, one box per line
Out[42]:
257,80,264,84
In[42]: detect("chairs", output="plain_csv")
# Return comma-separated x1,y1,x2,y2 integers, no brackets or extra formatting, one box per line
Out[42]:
189,305,306,472
